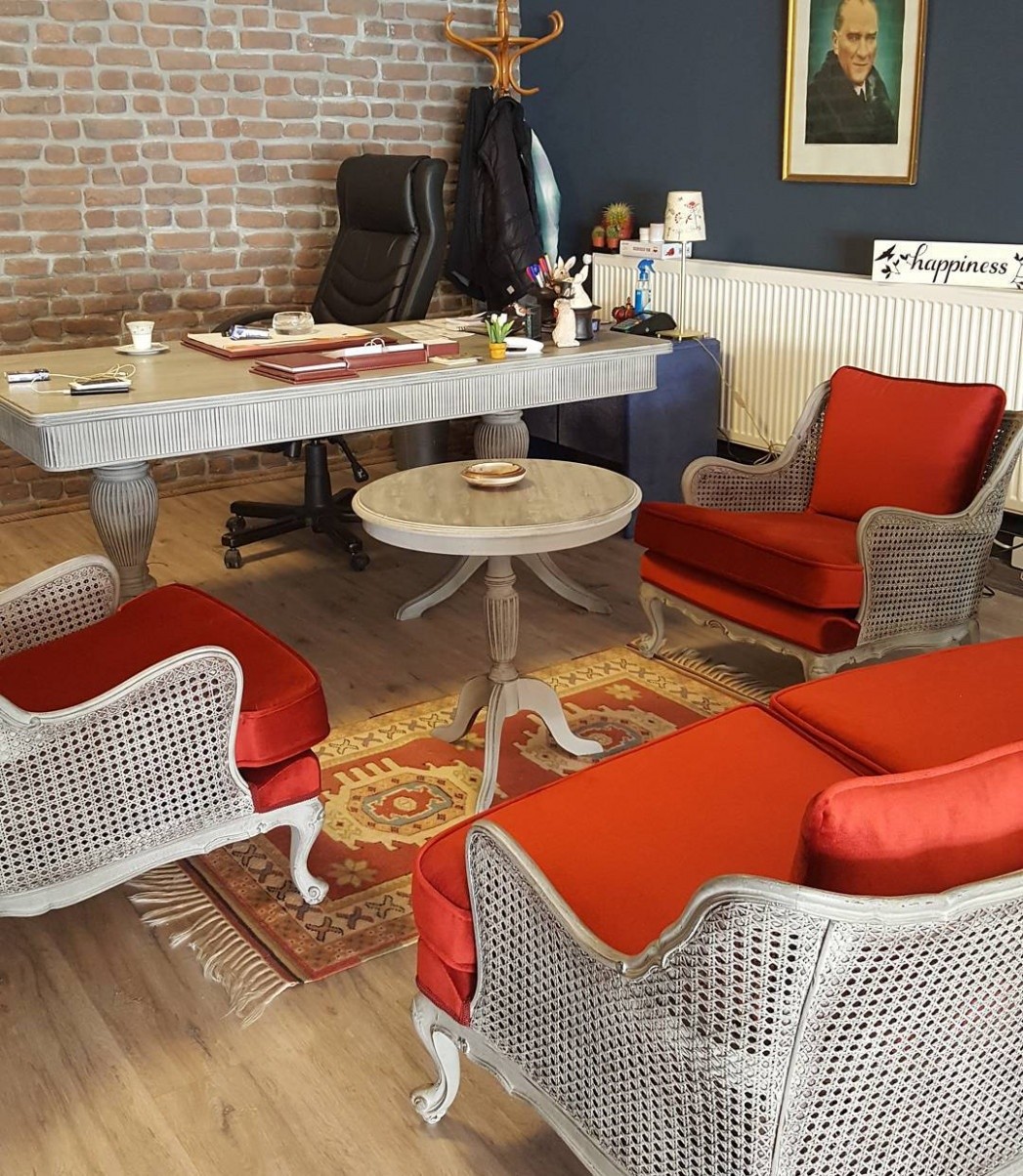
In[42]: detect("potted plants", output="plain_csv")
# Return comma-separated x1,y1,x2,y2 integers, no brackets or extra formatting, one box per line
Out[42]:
592,202,632,248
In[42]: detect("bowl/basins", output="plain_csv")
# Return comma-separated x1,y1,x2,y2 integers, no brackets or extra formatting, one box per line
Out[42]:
462,462,526,487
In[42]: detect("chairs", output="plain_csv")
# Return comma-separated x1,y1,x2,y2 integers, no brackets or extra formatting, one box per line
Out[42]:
634,366,1023,679
0,555,332,920
211,154,448,570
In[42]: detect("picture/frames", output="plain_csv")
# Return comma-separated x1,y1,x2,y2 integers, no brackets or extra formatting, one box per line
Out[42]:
782,0,929,186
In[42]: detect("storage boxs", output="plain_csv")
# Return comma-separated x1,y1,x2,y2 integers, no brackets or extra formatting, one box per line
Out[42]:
619,239,692,259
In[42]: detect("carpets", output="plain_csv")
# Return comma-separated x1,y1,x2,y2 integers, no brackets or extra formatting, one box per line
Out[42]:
117,634,785,1028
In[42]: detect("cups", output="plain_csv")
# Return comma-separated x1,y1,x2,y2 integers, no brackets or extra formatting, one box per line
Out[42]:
650,223,664,241
126,321,155,351
639,227,649,241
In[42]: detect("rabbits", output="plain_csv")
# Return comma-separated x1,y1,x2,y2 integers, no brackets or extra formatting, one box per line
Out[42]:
550,255,595,308
551,298,581,348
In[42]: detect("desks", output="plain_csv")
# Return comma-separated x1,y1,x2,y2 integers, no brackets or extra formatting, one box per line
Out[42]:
0,311,725,621
352,459,642,815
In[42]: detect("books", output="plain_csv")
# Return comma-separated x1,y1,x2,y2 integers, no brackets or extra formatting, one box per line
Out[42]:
255,352,347,374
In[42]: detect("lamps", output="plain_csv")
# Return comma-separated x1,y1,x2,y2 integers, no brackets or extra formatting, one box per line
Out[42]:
665,191,706,341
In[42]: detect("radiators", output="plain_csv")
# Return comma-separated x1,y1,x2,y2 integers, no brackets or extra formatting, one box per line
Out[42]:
593,253,1023,516
593,255,1023,517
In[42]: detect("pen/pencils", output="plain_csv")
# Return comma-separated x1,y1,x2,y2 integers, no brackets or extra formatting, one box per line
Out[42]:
527,253,552,288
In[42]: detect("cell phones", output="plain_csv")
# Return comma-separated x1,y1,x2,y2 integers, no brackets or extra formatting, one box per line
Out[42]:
67,376,132,391
4,368,51,383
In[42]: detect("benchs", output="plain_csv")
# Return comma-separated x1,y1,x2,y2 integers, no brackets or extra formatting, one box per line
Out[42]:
413,640,1023,1176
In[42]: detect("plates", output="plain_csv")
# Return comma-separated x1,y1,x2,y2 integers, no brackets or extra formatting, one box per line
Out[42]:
116,343,169,355
